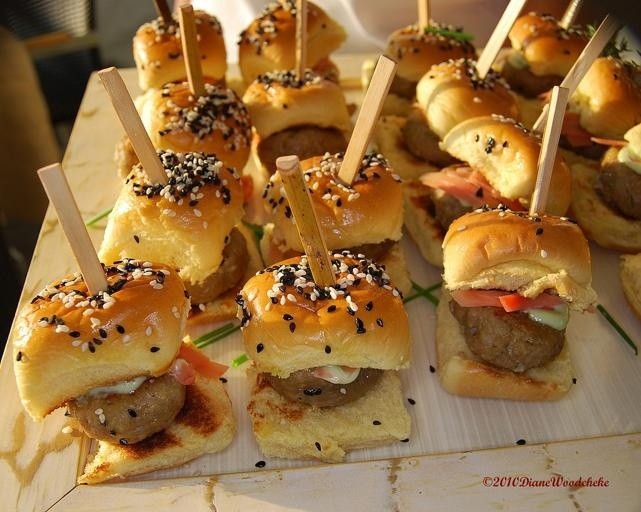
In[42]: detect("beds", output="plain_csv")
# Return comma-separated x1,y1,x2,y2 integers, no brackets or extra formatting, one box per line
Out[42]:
1,47,641,511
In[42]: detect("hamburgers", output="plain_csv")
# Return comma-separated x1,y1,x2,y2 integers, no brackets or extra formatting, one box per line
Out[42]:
10,0,641,487
